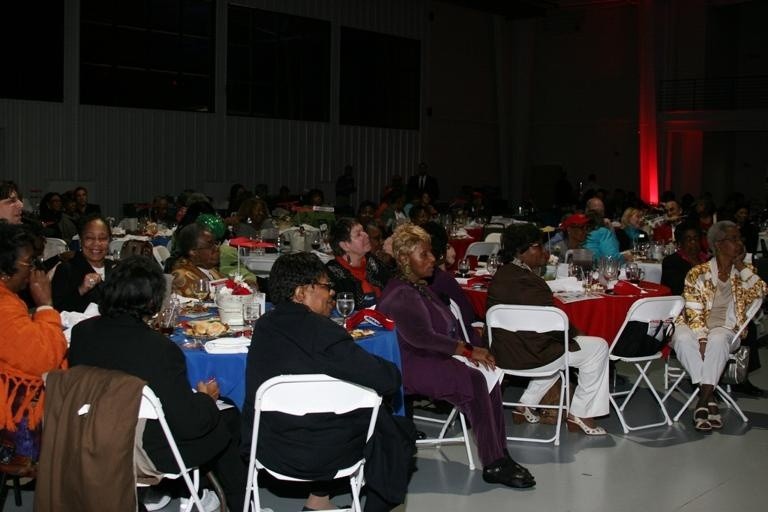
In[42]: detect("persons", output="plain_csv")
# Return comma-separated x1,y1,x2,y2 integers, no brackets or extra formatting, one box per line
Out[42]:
486,189,767,436
0,162,536,511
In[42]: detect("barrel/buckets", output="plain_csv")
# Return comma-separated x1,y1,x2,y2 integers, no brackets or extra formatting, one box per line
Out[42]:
213,292,254,327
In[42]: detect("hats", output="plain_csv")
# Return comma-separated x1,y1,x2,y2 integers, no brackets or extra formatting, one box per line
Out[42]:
559,212,590,230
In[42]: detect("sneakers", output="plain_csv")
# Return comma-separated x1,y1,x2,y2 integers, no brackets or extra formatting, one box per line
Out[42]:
200,488,221,512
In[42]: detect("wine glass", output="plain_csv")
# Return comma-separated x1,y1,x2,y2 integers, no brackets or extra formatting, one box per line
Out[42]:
602,259,618,296
335,291,355,329
450,224,458,238
310,231,320,252
242,302,261,338
457,257,472,276
273,237,284,257
487,257,499,277
192,278,210,306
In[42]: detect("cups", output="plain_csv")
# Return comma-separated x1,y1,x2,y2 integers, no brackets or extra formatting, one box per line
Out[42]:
625,261,646,284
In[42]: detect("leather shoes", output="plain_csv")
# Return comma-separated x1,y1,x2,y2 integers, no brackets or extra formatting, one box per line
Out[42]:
481,463,537,488
505,450,532,481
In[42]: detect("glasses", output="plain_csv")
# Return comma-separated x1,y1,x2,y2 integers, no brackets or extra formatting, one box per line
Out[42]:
720,234,744,245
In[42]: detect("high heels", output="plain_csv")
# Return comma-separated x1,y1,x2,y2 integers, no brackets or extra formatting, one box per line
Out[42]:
566,416,608,436
512,406,541,424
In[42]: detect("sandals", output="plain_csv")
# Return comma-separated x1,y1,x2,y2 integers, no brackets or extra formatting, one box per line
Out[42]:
707,401,724,429
693,407,713,431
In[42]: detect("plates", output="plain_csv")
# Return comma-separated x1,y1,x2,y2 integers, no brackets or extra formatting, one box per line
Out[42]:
182,331,233,338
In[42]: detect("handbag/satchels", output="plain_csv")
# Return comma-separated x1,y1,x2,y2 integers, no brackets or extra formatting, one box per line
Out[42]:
611,320,669,358
715,345,751,385
